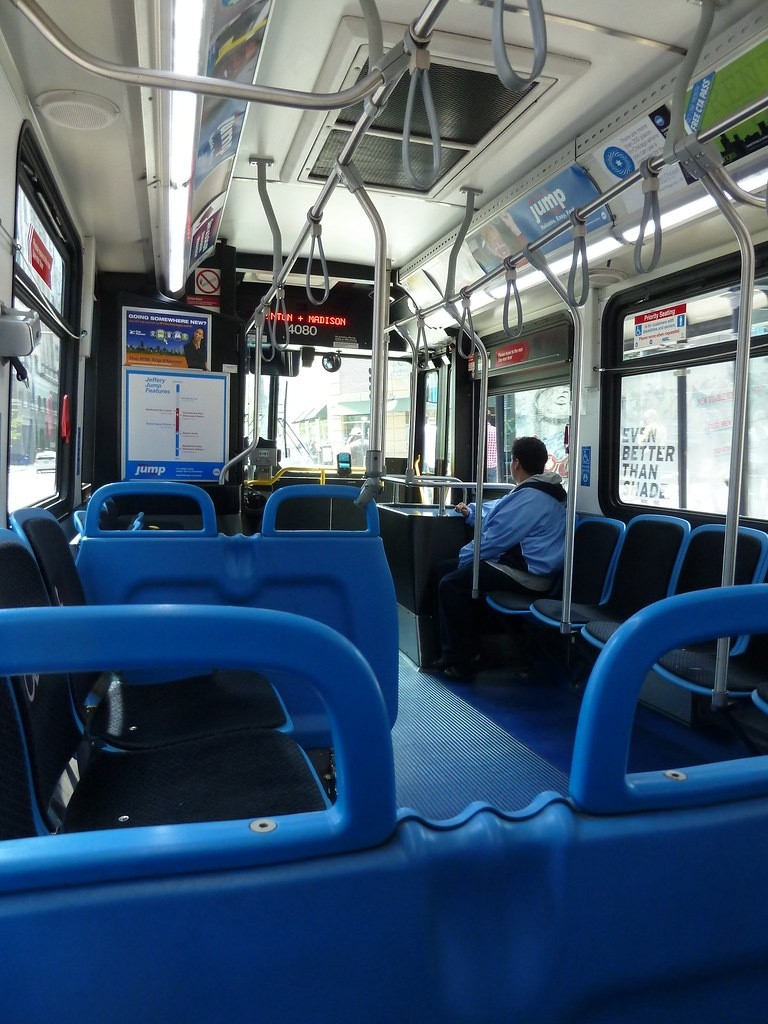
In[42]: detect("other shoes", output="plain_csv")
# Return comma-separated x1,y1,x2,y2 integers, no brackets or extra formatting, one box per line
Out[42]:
431,654,454,670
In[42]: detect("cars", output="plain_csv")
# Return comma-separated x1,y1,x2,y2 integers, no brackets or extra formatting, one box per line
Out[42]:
34,451,56,474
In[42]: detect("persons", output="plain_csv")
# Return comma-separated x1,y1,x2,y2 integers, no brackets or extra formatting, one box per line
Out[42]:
201,37,261,123
486,411,498,483
184,327,207,372
480,211,532,278
342,427,369,467
290,440,318,467
421,436,568,679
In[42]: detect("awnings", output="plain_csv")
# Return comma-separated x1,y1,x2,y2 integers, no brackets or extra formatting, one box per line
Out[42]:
290,405,327,424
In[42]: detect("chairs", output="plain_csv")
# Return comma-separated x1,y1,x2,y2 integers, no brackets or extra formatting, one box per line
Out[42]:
0,508,331,837
486,513,768,716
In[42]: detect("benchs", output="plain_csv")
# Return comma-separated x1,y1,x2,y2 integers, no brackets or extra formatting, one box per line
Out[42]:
75,480,400,752
1,582,768,1022
